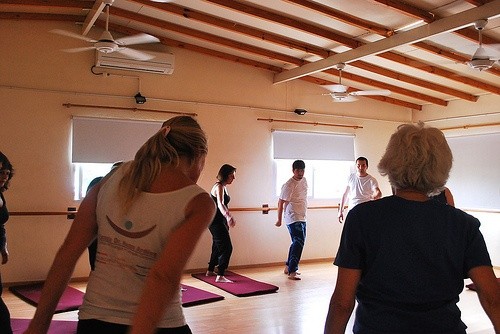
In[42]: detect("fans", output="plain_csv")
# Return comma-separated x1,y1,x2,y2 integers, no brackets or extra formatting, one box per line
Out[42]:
52,1,161,61
302,63,391,103
410,19,500,76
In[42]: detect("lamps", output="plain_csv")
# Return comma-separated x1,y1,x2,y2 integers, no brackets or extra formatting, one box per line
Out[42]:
295,109,307,115
135,96,146,104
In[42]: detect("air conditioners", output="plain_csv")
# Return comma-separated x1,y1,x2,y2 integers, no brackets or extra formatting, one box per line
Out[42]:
94,49,175,75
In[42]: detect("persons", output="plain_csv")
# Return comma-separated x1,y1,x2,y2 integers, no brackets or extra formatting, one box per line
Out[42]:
23,116,217,334
206,164,236,284
0,152,13,334
275,160,308,280
338,157,382,224
324,123,500,334
